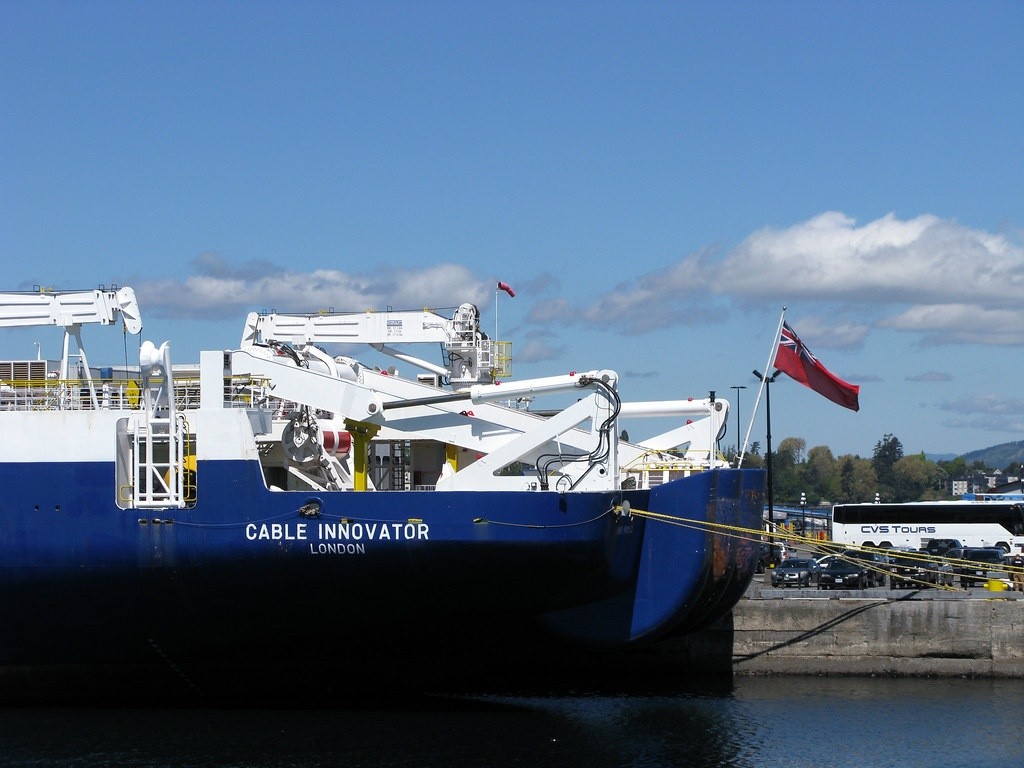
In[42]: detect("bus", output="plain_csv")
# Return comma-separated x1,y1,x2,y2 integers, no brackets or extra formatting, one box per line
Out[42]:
832,500,1024,565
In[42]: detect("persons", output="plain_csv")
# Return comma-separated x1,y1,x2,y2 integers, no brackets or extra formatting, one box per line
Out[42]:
1009,554,1024,592
937,554,972,590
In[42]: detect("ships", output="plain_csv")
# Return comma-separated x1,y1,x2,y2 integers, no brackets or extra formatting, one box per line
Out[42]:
0,282,770,661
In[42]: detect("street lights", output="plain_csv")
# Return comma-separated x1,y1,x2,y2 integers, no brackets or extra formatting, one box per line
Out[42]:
730,386,747,457
800,491,808,537
752,367,781,558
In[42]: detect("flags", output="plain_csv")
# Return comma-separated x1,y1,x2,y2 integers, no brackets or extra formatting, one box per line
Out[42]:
498,281,516,297
771,319,860,412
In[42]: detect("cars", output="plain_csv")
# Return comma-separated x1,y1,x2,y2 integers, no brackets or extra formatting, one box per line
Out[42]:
811,538,1024,590
770,558,819,588
755,543,781,572
